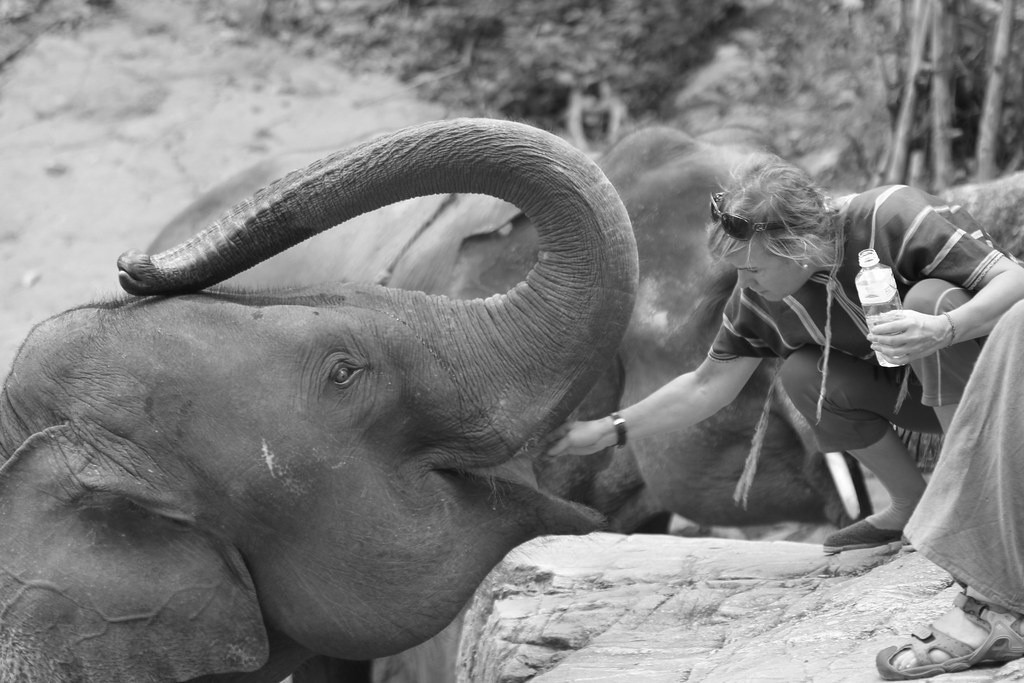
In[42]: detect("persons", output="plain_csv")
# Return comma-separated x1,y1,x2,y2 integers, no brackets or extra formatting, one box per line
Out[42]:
874,298,1024,679
530,154,1023,551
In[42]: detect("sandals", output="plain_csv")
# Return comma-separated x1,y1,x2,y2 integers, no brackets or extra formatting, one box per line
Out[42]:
877,591,1024,680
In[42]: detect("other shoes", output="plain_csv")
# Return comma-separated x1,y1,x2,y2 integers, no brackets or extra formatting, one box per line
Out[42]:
822,520,903,553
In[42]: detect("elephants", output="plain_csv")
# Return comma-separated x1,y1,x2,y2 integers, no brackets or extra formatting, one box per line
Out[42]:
142,122,876,536
1,116,641,683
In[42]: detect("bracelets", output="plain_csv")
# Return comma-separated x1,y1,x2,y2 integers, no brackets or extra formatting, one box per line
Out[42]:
613,412,626,448
944,312,955,348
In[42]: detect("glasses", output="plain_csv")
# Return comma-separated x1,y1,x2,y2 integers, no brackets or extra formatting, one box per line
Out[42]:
709,192,796,239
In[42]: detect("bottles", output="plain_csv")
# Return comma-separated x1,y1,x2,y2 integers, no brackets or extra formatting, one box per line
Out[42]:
854,247,913,369
